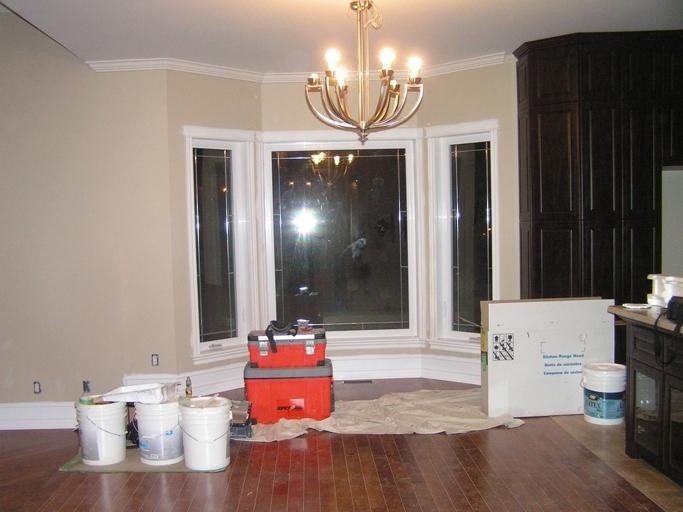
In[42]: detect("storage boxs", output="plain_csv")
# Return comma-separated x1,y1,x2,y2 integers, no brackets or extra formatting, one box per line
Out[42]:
248,329,327,367
244,359,335,422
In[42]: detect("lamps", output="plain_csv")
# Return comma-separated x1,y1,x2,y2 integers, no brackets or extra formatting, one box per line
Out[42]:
308,150,355,187
304,0,425,143
308,151,354,186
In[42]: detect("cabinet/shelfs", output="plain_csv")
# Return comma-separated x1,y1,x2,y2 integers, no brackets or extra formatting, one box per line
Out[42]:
512,29,683,305
607,304,683,487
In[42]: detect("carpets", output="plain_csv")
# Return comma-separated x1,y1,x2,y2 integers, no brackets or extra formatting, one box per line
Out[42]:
230,388,525,443
57,440,227,474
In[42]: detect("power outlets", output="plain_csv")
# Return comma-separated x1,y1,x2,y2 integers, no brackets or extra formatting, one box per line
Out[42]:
33,381,41,394
152,353,158,367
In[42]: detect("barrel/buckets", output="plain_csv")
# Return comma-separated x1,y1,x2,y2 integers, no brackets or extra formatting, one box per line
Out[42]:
579,362,626,426
125,402,137,450
179,396,234,471
73,394,130,466
131,400,184,466
